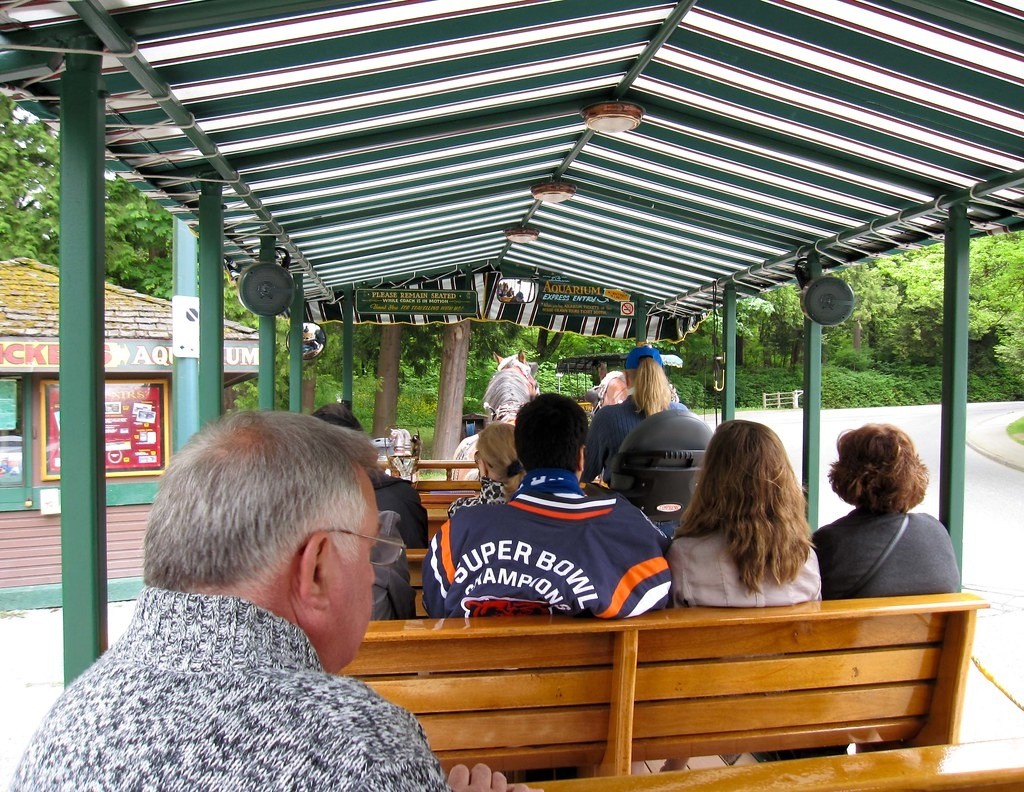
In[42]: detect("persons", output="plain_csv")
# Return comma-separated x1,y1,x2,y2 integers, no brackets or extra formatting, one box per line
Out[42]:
369,510,417,622
811,423,960,600
447,422,526,518
578,345,688,483
499,282,524,302
9,407,546,792
421,392,672,619
667,420,823,608
313,403,430,548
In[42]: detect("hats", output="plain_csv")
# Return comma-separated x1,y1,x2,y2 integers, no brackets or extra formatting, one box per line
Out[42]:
625,346,663,369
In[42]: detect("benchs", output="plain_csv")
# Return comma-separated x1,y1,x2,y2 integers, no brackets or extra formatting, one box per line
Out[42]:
328,458,1024,792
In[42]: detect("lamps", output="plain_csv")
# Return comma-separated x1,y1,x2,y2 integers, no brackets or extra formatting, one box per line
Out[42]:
505,226,540,243
530,182,578,204
579,101,646,134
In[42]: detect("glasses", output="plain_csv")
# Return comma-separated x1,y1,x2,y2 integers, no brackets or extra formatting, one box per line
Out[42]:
301,511,405,565
474,451,493,468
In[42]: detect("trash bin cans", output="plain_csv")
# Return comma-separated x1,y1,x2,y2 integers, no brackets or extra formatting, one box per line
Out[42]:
370,438,394,456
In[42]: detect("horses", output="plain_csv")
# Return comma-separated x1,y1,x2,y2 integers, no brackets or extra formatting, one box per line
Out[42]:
585,370,631,406
451,349,540,482
389,428,413,456
